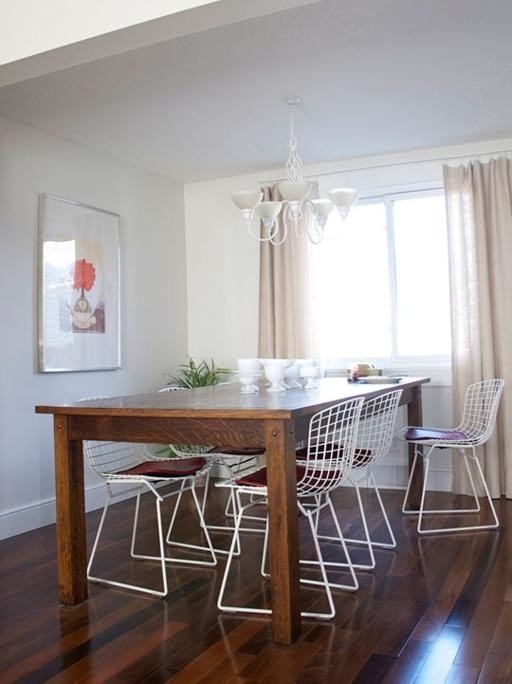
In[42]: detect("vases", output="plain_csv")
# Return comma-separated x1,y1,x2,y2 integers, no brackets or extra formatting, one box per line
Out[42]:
72,296,91,329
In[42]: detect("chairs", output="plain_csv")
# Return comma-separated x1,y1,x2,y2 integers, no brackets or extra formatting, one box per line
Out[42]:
295,389,404,570
69,396,217,597
156,387,264,559
217,397,365,618
219,454,267,522
396,379,505,534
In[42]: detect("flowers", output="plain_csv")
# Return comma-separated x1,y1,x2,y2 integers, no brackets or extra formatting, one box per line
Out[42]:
68,259,96,296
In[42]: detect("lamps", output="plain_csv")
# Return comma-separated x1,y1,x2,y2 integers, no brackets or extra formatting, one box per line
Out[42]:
232,95,358,244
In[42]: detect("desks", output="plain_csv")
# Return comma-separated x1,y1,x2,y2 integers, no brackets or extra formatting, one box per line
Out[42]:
36,377,430,645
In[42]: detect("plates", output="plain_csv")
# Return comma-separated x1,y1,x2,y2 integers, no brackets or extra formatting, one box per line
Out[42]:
359,375,402,384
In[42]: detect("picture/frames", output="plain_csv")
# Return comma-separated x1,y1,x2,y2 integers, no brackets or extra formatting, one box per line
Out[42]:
37,195,122,371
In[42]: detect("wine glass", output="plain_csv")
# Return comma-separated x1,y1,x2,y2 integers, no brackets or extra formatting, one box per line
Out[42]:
239,357,320,395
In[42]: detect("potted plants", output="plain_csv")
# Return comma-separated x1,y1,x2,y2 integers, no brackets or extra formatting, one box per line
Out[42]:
156,358,231,487
